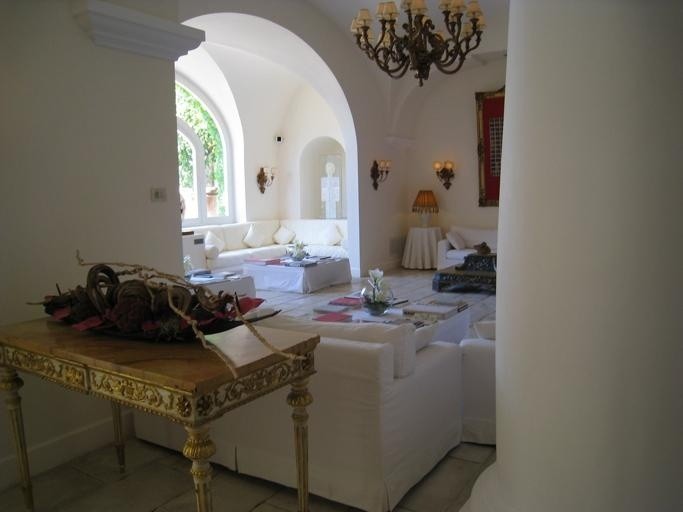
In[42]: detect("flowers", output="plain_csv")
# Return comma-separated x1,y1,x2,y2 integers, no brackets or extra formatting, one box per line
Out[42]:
363,268,387,303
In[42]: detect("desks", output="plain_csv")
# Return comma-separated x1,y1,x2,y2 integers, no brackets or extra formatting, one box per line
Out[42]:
401,226,442,270
1,315,319,511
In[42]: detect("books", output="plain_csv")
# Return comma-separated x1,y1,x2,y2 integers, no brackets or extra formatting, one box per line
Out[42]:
243,254,348,269
311,288,470,328
189,268,237,287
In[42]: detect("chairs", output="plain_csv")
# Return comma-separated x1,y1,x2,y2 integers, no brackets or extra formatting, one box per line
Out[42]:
459,336,496,445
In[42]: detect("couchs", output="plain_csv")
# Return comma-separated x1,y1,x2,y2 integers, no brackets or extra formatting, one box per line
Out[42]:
186,218,347,272
133,277,462,511
437,227,497,271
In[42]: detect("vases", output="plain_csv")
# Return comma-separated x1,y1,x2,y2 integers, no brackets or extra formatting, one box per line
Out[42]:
360,282,393,315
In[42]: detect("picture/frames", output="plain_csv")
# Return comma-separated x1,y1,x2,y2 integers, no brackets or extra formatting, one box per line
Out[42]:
475,84,505,206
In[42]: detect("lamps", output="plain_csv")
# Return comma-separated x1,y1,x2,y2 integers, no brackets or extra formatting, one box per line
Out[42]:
257,167,277,194
412,190,439,227
370,160,391,191
349,0,486,87
433,160,455,190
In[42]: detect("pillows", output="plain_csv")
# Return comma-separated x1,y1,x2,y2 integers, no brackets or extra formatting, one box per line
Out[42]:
444,231,466,250
474,322,494,341
205,223,342,260
234,297,433,380
